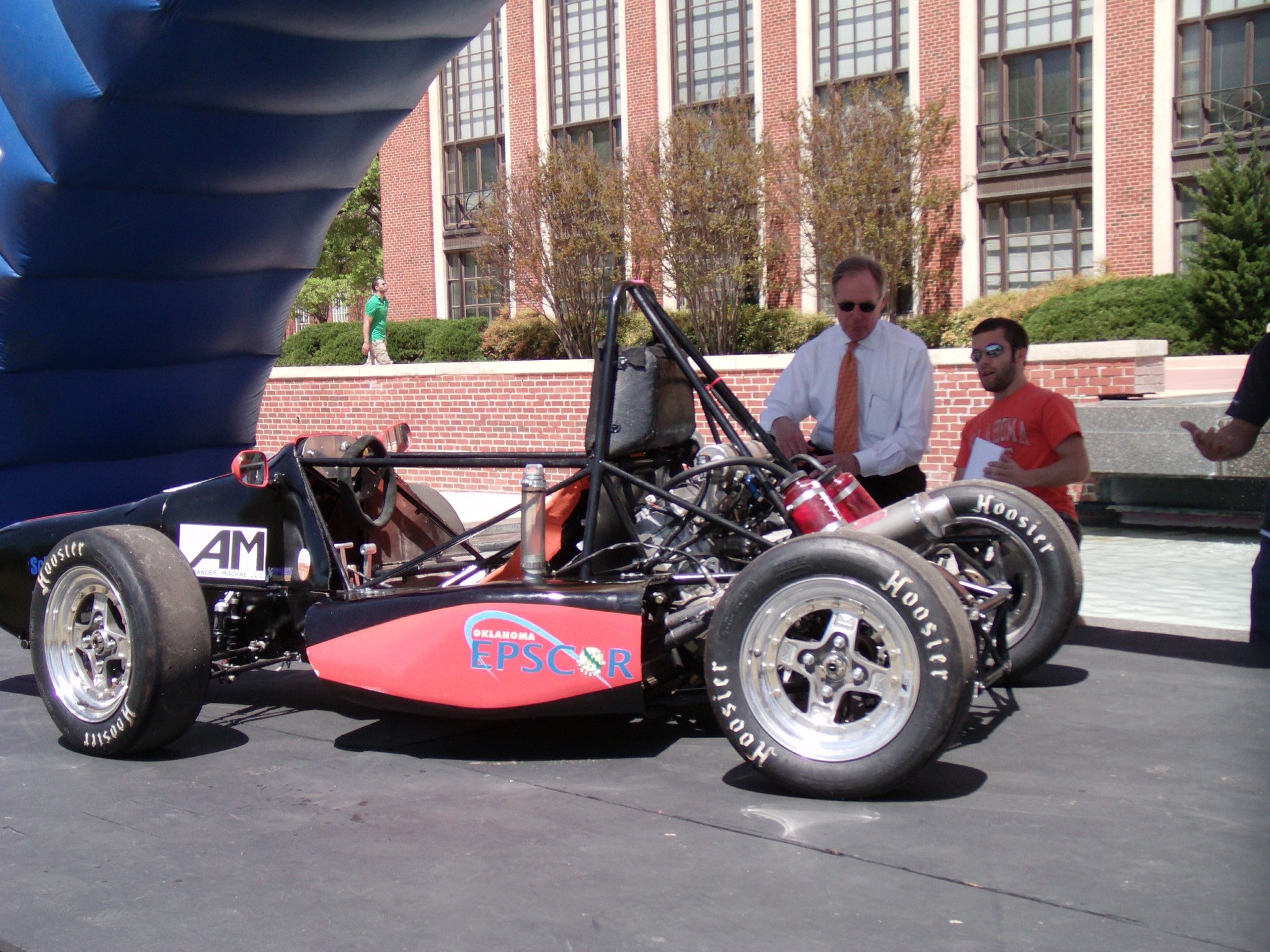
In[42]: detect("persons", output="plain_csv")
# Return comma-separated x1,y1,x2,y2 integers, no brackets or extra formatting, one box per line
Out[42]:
361,277,393,365
1179,326,1270,650
759,255,932,512
950,318,1091,549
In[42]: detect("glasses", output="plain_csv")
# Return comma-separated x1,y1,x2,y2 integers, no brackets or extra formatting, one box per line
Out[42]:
838,301,877,313
971,343,1014,363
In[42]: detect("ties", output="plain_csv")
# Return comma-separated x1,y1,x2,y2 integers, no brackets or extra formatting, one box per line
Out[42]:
834,341,858,454
